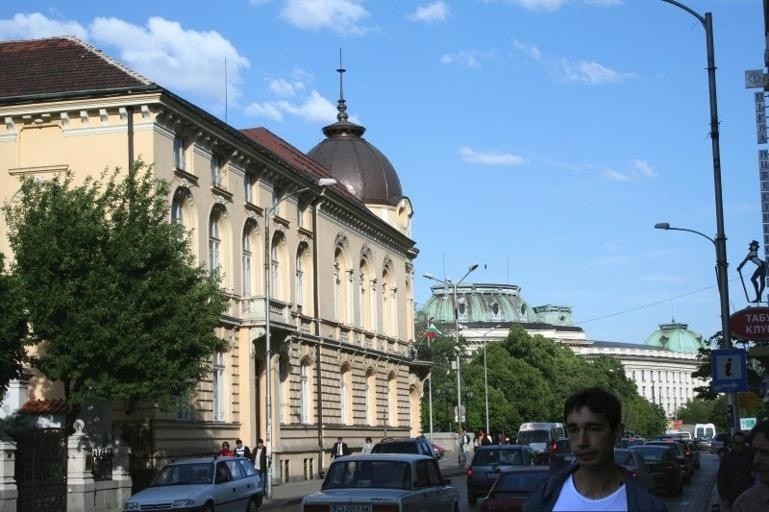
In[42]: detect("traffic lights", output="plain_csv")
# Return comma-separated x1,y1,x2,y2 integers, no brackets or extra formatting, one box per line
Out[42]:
726,404,735,428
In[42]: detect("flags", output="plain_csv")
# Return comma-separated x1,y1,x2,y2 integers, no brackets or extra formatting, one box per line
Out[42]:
423,324,442,338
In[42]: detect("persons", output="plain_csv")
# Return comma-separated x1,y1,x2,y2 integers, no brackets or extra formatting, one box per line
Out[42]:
218,441,233,457
462,429,514,462
738,240,767,303
251,439,266,494
732,418,769,512
361,437,374,454
691,432,694,436
521,386,667,512
331,437,348,459
234,440,249,457
717,431,755,508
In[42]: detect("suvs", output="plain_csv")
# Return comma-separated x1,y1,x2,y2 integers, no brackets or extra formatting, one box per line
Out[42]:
370,435,436,459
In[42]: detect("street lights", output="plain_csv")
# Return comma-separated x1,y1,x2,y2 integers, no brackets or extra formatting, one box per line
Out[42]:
264,177,337,494
423,262,482,436
426,314,434,443
654,223,739,443
484,324,502,434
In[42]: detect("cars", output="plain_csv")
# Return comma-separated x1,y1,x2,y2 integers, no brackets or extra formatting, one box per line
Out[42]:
126,455,262,512
466,421,716,512
429,442,445,458
304,453,460,511
